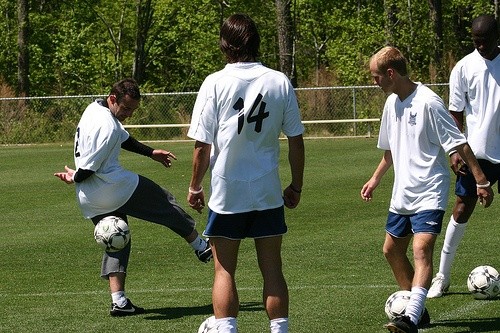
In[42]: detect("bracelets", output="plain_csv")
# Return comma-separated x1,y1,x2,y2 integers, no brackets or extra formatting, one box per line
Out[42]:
290,184,301,193
189,185,203,194
449,150,457,157
477,181,490,188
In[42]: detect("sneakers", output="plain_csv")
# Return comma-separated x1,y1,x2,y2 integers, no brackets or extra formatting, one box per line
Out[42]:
425,271,451,298
109,298,145,316
387,315,418,333
198,316,239,333
415,306,430,329
194,238,215,263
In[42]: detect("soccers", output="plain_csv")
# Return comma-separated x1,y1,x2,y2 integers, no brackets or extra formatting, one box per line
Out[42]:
198,315,215,333
467,265,500,299
94,215,130,252
385,290,425,324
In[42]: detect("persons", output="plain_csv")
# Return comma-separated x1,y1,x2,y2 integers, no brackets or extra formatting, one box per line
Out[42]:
187,13,306,333
53,80,213,317
426,16,500,297
361,46,495,333
